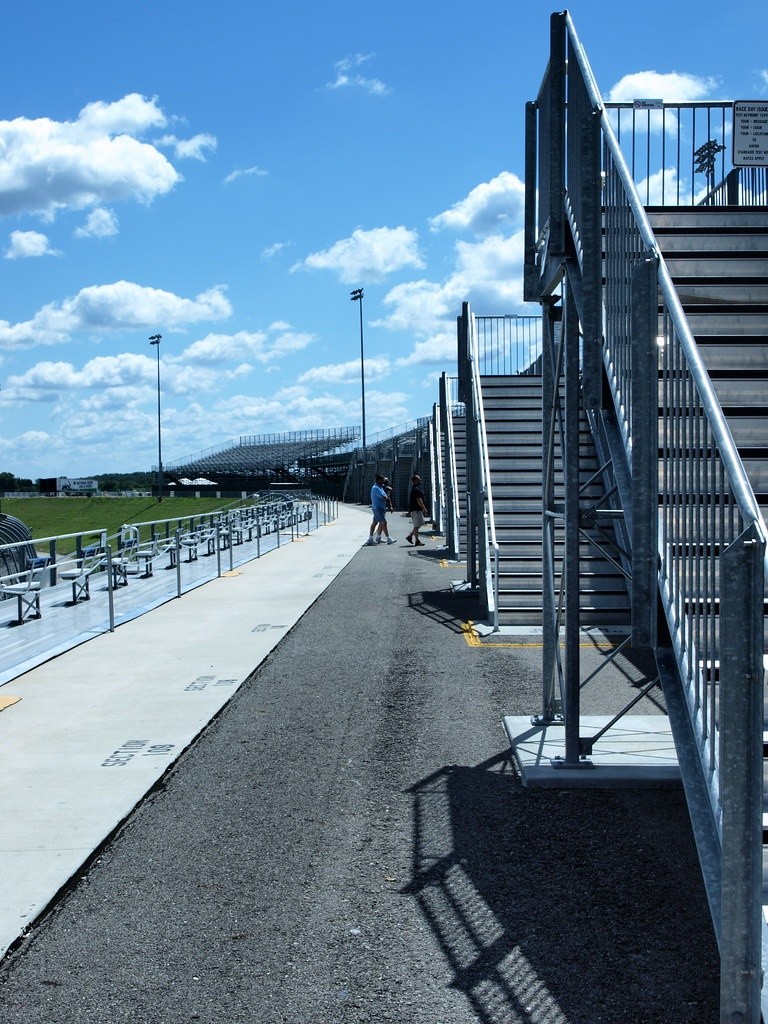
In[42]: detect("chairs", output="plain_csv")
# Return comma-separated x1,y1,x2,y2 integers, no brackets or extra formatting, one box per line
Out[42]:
3,556,52,625
57,546,101,604
101,505,306,591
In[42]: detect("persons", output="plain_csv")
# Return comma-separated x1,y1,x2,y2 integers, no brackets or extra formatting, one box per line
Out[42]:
406,475,430,546
366,473,397,545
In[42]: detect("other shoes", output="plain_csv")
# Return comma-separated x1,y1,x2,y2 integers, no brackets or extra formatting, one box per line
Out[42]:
375,537,386,543
415,540,425,546
388,537,397,545
367,538,378,545
406,536,413,544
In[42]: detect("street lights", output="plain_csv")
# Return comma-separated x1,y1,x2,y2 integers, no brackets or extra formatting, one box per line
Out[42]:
692,139,725,205
351,287,366,448
149,334,163,497
